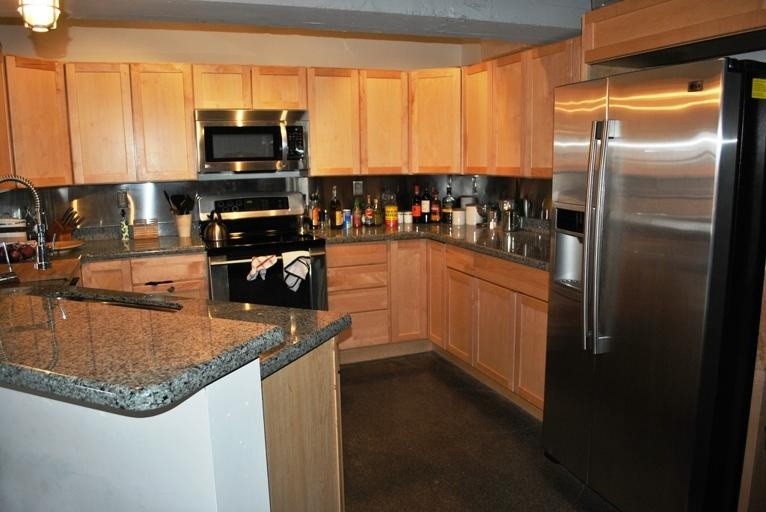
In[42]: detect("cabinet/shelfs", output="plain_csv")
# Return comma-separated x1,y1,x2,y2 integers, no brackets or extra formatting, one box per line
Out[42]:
527,33,648,181
192,64,310,112
425,238,544,413
62,58,198,186
128,256,208,298
461,47,528,179
0,52,17,192
307,66,411,179
185,299,360,512
1,51,75,193
326,241,427,352
411,65,463,176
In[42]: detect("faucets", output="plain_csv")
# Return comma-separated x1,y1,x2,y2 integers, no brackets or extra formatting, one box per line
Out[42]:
0,174,57,271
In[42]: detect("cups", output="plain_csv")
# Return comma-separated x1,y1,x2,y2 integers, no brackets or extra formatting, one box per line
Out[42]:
175,214,192,239
466,225,482,244
482,195,549,232
178,238,193,247
466,204,484,225
452,224,465,239
484,234,549,258
452,209,466,225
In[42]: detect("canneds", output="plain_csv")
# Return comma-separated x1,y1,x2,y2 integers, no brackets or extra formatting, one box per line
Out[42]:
398,223,412,232
398,211,413,224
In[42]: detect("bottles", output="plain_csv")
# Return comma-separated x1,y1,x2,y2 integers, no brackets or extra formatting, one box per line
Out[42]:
307,180,455,228
121,240,130,250
119,209,130,240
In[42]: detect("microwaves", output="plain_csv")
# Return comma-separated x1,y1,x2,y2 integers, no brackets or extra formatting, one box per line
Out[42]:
194,121,310,171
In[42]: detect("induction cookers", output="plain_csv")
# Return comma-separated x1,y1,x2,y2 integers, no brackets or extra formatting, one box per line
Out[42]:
195,220,324,259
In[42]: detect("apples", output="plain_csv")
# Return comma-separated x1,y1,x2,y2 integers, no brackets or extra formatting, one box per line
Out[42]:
0,243,35,261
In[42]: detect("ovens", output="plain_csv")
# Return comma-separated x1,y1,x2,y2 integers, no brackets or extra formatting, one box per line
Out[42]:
207,247,327,312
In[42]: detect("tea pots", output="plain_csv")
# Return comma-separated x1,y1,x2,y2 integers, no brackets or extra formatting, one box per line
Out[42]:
202,210,230,240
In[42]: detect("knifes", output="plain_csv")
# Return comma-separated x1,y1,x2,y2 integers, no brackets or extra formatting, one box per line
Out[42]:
60,206,85,226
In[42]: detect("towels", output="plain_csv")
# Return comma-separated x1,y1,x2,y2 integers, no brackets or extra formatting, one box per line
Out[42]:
278,247,315,296
244,249,279,283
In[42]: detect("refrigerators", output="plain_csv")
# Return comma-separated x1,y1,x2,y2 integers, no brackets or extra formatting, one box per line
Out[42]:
540,55,766,511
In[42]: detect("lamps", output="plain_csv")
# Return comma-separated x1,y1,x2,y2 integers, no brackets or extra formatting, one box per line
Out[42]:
14,1,64,36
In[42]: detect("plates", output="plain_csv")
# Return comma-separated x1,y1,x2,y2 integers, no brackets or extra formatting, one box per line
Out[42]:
42,238,86,250
